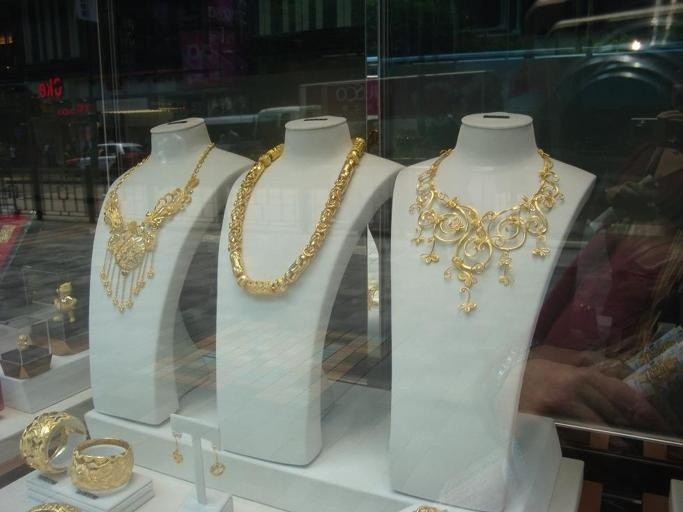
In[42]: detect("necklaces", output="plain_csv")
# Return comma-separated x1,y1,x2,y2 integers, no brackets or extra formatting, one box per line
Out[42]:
227,136,366,296
100,140,217,312
409,144,565,313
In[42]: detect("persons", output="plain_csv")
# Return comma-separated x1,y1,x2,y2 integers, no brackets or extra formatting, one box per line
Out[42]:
582,109,683,229
517,230,682,441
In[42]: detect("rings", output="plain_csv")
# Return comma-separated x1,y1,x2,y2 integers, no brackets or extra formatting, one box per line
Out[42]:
18,334,30,350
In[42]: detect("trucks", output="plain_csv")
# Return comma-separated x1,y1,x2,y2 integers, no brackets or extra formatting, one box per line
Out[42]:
203,104,322,160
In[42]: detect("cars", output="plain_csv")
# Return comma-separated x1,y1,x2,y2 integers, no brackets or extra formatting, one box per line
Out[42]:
66,143,150,181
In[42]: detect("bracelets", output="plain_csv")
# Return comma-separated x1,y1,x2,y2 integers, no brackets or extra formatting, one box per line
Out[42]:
71,436,133,494
19,413,90,478
